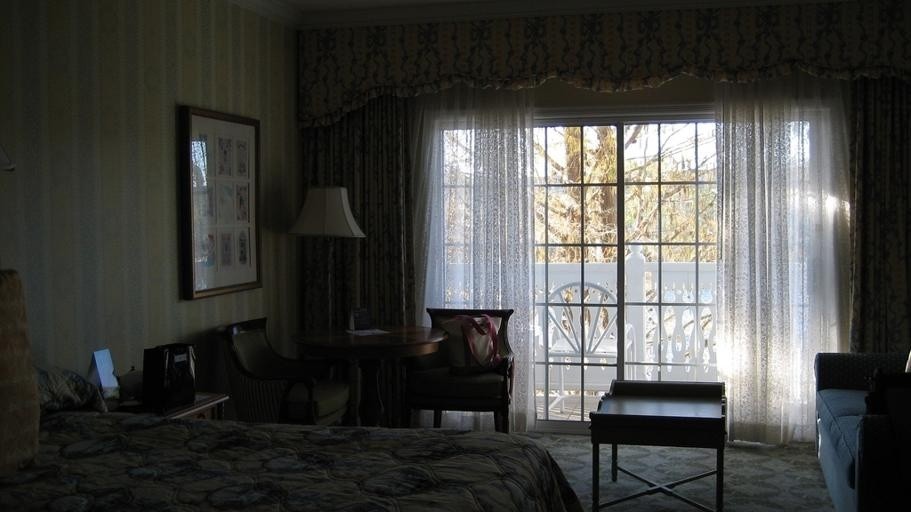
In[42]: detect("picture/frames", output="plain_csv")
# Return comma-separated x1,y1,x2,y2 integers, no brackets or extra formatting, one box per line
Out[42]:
176,105,264,301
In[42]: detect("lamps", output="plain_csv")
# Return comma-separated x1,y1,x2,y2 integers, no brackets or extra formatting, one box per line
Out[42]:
289,186,366,336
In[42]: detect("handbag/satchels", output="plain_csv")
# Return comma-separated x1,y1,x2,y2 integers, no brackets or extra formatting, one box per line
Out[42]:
439,312,502,373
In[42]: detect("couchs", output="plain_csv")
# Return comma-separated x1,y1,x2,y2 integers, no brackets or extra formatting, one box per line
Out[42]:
815,351,911,512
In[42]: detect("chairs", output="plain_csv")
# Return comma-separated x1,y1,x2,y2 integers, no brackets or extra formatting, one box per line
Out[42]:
209,316,368,425
646,302,716,379
401,308,513,432
544,281,633,420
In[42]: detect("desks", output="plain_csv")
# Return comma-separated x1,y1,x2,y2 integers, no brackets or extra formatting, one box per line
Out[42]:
590,380,727,512
293,326,449,428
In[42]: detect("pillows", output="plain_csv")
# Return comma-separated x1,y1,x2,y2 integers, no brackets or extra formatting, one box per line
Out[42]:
864,359,911,414
229,332,283,380
0,269,40,479
36,366,108,422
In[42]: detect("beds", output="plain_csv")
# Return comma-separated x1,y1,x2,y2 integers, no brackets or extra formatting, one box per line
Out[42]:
1,410,581,512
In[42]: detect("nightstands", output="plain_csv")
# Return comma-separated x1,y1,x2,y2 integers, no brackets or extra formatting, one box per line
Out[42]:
110,388,229,421
